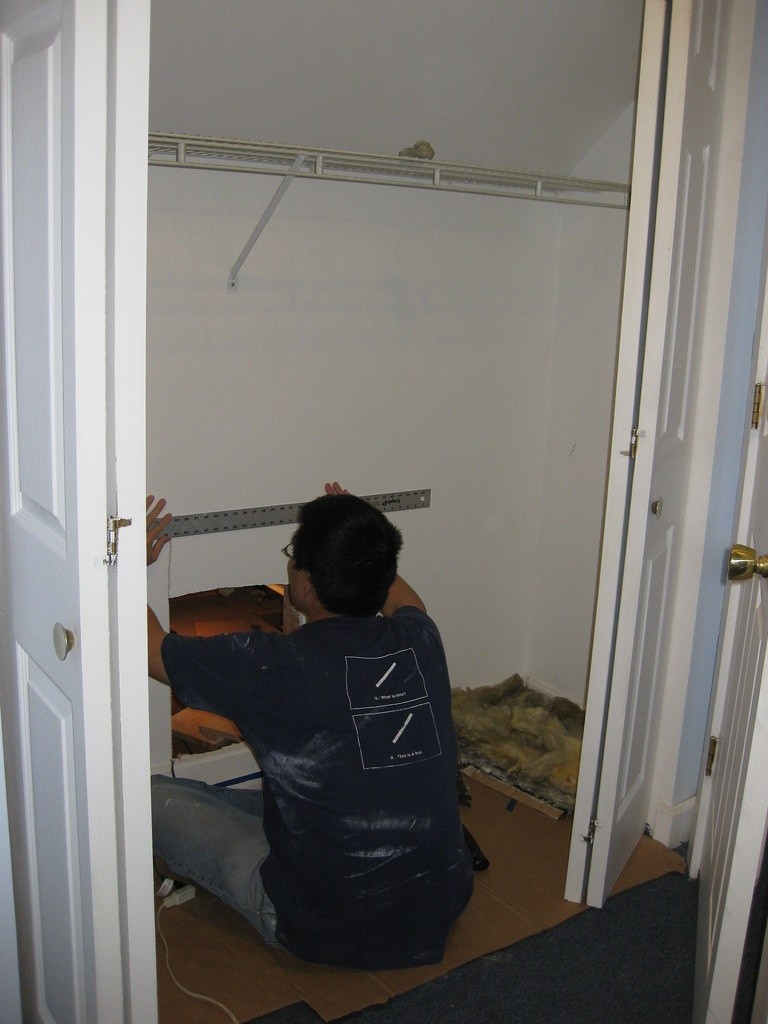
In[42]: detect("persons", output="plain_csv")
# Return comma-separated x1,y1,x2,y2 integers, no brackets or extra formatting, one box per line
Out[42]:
146,483,475,970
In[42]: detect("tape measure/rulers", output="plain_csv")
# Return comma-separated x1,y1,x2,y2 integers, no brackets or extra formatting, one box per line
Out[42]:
148,488,430,538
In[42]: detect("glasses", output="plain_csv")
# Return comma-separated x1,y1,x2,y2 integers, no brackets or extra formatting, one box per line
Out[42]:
281,542,295,560
